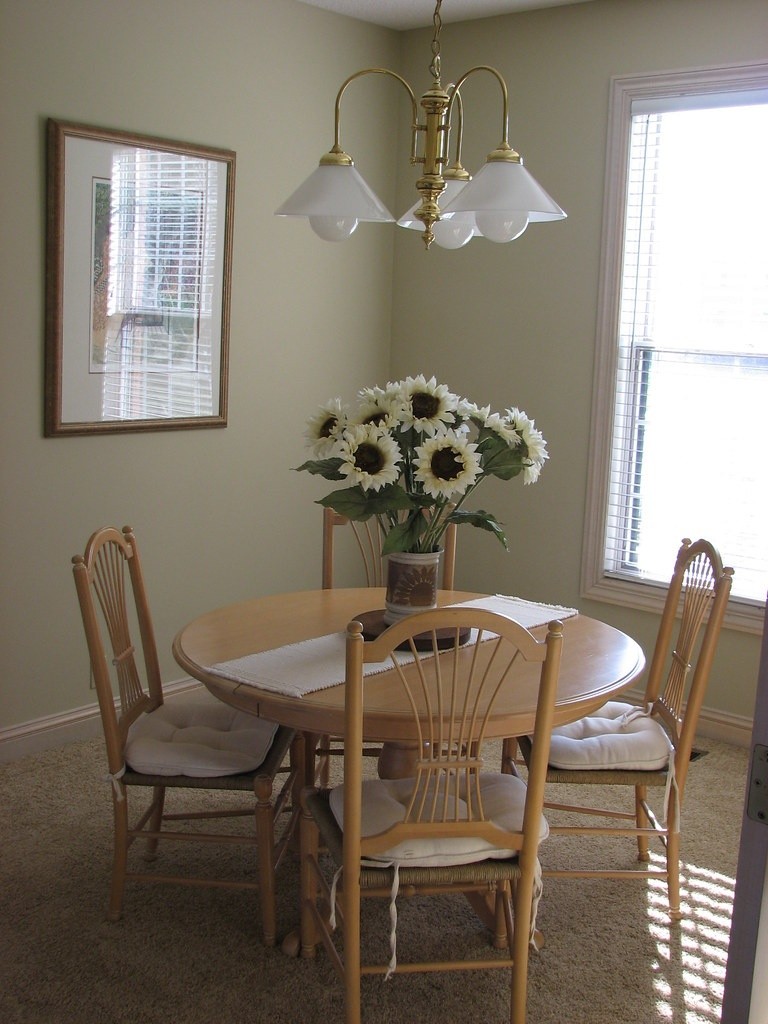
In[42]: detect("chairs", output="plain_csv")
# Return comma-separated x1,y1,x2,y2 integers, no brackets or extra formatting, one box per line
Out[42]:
297,601,572,1021
66,523,323,950
489,534,737,932
316,496,461,594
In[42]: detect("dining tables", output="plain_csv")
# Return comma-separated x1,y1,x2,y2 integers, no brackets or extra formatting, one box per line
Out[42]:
169,583,652,785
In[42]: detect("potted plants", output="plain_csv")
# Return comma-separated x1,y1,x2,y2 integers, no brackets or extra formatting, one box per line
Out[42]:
381,541,451,632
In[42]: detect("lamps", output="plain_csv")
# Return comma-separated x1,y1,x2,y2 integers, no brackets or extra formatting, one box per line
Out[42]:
272,1,567,253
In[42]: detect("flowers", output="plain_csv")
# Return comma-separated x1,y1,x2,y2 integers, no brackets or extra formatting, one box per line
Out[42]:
295,375,551,551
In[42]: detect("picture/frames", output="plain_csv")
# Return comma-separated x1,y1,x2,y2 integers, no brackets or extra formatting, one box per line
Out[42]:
42,113,237,437
